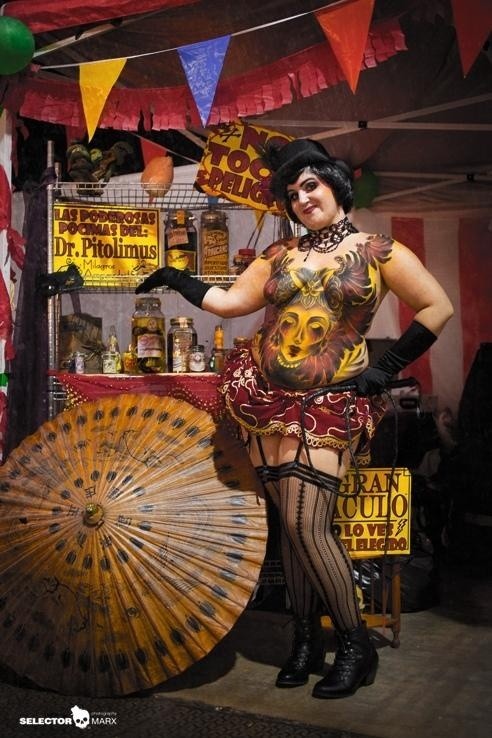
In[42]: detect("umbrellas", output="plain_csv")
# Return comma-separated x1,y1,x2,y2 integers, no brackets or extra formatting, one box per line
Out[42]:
0,389,271,700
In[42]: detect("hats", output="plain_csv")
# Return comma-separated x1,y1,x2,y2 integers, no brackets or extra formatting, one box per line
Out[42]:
267,139,355,197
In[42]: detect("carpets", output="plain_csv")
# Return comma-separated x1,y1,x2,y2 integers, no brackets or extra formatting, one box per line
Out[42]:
0,684,380,738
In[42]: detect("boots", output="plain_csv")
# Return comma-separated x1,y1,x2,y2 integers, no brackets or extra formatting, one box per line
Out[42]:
275,613,327,688
314,619,380,698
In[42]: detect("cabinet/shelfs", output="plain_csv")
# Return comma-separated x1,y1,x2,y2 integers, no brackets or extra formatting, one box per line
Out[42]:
46,182,301,421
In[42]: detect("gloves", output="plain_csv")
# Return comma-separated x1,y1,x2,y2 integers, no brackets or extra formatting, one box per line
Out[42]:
135,266,212,309
340,319,437,399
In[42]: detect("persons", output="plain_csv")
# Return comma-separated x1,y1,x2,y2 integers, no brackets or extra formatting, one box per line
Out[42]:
133,139,454,701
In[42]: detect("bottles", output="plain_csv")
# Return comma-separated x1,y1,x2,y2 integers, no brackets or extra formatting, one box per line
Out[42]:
231,248,257,280
168,316,206,373
210,326,227,371
163,211,197,281
200,212,230,281
102,326,121,373
124,296,166,373
